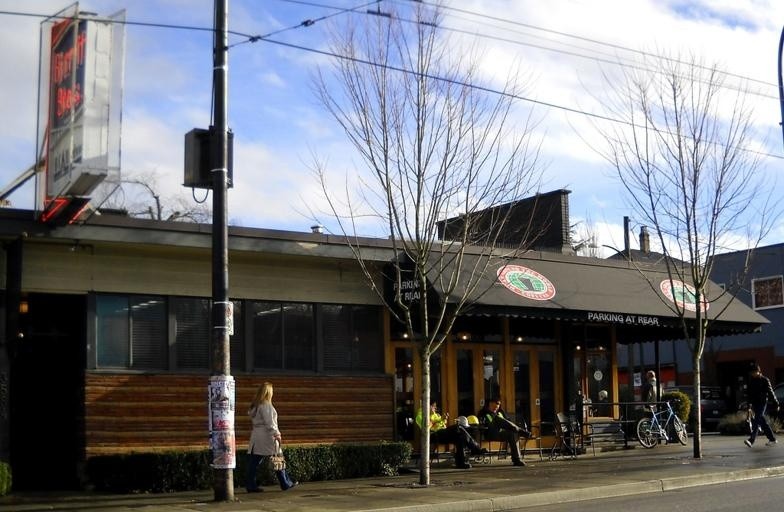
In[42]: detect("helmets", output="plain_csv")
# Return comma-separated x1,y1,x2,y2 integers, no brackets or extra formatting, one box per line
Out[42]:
599,390,608,401
454,416,470,429
467,415,479,425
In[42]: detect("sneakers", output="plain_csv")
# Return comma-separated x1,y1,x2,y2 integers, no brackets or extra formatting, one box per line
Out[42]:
745,440,752,448
766,440,778,446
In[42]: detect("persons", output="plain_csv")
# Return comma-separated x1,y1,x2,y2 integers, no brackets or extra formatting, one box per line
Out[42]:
414,400,490,469
476,396,537,467
242,380,300,494
743,362,781,448
643,368,666,415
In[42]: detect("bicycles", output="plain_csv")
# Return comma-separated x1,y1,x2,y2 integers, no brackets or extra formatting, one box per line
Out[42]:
636,400,688,449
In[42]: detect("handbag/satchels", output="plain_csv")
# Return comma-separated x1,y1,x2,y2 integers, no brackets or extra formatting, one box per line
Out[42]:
745,410,762,436
269,437,288,473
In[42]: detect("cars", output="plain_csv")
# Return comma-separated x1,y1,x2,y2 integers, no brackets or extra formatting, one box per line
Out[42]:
738,384,784,419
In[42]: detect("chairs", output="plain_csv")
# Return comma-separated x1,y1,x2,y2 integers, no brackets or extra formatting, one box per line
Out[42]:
415,410,595,468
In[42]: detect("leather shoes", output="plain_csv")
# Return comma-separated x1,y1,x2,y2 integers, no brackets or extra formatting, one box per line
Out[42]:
514,460,527,466
456,463,472,469
522,430,532,439
248,488,264,493
289,481,299,489
471,448,486,456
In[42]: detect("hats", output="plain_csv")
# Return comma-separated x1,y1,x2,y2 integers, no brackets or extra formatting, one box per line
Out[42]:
646,371,656,382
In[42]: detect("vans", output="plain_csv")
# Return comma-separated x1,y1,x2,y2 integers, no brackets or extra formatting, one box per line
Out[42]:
679,385,725,415
